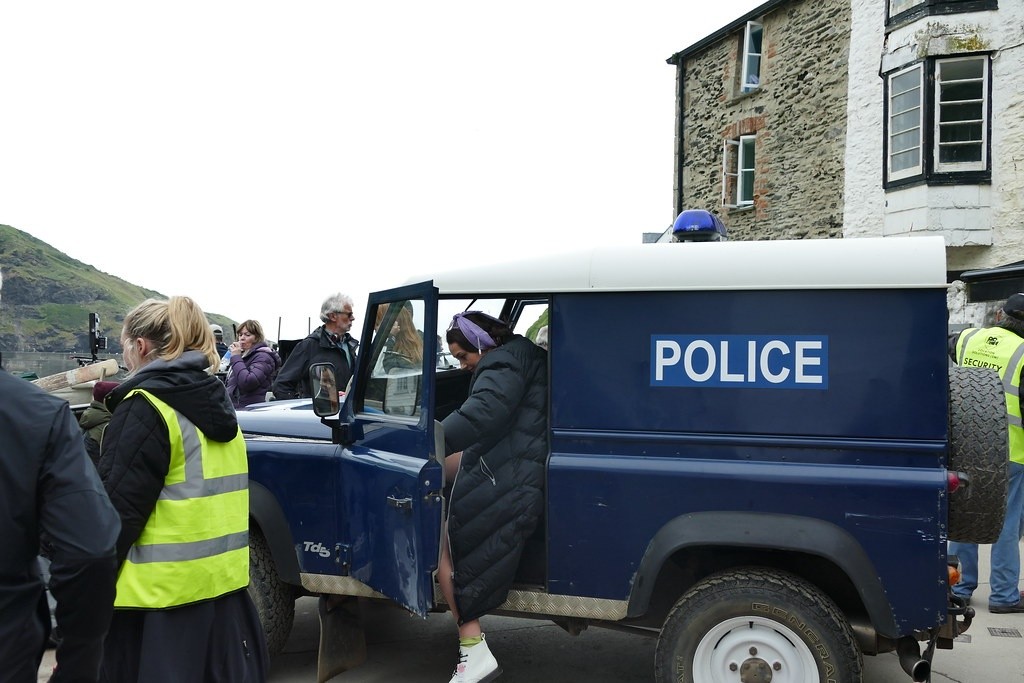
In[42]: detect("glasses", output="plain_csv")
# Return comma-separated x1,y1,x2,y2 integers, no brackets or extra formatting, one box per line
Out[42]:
336,311,353,318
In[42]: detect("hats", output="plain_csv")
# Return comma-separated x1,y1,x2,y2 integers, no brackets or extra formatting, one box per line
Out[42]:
1003,293,1023,320
92,382,118,403
210,324,223,335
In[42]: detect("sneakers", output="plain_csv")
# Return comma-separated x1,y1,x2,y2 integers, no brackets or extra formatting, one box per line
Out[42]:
449,632,503,683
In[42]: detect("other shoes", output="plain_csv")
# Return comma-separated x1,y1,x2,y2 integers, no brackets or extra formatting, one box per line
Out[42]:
989,594,1024,614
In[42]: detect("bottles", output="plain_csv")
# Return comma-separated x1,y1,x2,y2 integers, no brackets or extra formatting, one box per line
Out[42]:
219,350,232,371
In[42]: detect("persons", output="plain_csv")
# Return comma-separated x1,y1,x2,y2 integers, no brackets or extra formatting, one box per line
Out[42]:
437,311,548,683
210,292,443,410
948,292,1024,614
0,295,251,683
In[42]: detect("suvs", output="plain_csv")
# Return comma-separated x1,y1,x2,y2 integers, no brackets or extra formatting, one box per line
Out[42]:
236,209,1009,683
